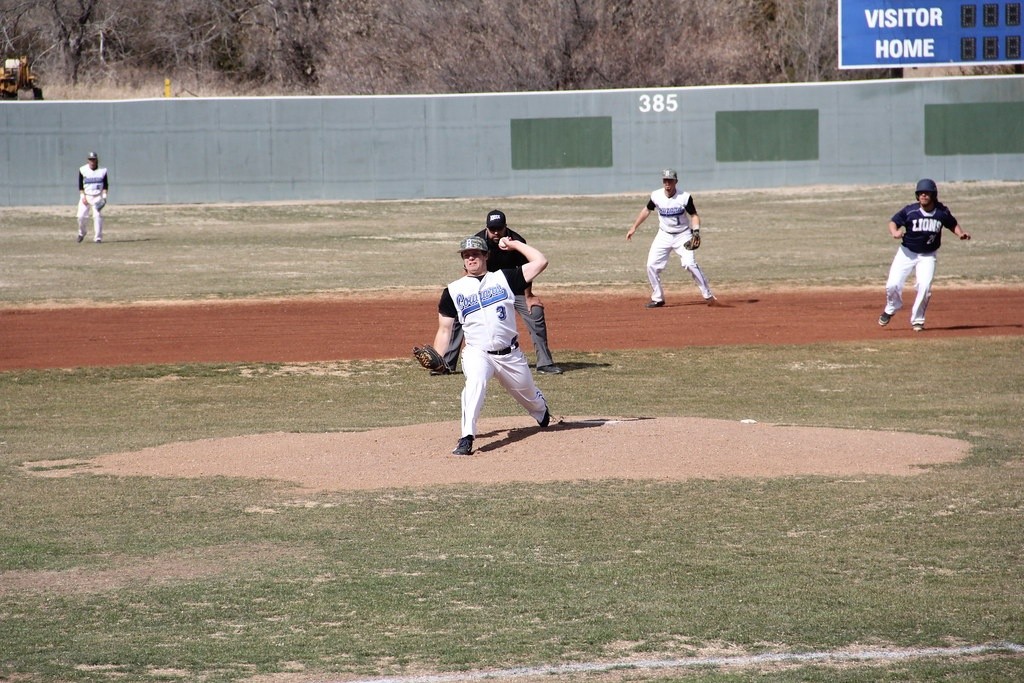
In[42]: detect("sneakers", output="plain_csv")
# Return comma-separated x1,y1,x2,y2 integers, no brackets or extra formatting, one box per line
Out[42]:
536,408,549,427
878,312,892,326
430,370,443,375
913,324,924,331
536,365,562,375
453,436,474,455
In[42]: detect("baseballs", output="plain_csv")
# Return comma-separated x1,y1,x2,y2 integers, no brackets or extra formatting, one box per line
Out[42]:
499,236,509,248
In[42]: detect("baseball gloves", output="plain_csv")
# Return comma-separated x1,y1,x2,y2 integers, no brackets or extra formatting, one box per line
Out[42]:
683,233,701,251
412,344,449,373
94,198,106,211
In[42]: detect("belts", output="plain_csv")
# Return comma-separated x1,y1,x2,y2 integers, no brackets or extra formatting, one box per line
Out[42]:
668,227,689,234
488,342,518,355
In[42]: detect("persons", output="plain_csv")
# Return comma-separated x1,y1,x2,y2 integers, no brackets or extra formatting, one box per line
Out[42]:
625,167,718,308
878,178,971,333
77,152,109,243
428,209,564,375
412,235,551,457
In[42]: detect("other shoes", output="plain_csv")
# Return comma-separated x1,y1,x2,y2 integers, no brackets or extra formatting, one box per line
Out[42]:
647,301,664,308
77,235,83,242
96,240,101,243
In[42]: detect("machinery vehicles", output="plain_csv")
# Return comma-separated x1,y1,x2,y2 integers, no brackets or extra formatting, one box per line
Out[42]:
0,56,43,101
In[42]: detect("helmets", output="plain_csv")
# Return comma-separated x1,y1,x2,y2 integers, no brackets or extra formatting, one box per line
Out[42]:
915,179,938,200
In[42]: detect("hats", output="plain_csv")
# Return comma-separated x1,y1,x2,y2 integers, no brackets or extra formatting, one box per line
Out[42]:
486,210,506,226
661,168,678,181
458,235,489,253
87,152,97,160
